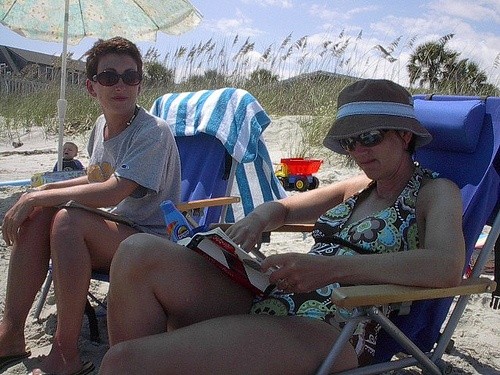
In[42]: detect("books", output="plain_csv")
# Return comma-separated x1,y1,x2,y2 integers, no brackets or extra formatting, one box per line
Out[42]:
177,227,274,296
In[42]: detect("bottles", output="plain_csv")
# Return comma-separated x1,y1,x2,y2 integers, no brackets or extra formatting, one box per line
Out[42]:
159,200,195,247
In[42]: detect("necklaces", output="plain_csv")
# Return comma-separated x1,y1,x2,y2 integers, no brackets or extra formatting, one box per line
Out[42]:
126,103,139,127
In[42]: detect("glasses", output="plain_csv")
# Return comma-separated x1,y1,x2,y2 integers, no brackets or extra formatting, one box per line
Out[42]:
92,70,143,87
339,129,388,153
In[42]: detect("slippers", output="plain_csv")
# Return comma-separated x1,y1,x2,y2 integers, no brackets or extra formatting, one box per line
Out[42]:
26,359,95,375
0,346,32,369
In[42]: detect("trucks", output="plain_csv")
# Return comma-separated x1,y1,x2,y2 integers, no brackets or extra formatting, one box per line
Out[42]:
274,158,323,192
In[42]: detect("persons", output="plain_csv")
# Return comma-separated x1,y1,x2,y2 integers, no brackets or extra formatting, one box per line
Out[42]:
0,38,180,375
53,142,84,172
96,80,466,374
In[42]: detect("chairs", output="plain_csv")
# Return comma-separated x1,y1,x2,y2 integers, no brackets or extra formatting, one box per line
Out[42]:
206,95,500,375
33,88,288,318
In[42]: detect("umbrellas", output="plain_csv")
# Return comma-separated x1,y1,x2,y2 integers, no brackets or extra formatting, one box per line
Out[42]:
0,1,203,172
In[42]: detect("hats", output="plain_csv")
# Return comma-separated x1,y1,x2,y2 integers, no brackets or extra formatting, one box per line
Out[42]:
323,79,433,155
